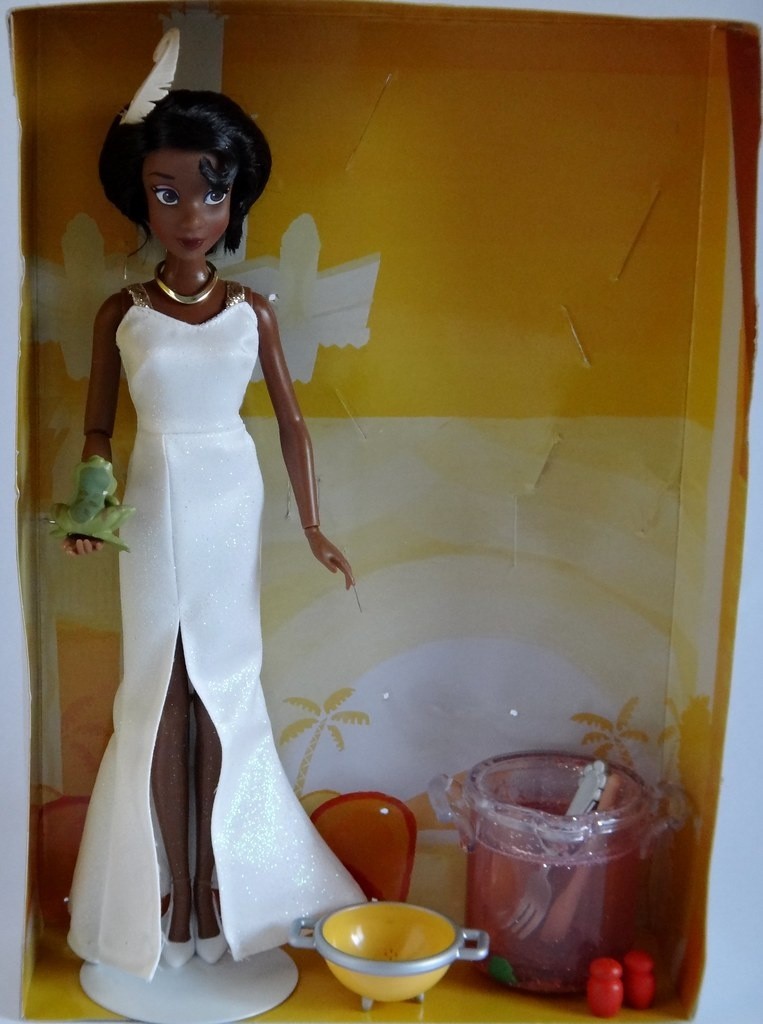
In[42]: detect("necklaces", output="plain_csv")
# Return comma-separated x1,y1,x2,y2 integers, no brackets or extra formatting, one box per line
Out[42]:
153,256,220,304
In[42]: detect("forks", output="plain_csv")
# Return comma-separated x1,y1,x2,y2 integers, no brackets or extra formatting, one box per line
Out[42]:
501,760,607,939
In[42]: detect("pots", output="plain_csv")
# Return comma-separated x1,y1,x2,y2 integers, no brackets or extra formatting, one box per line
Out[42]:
289,903,489,1012
426,750,691,992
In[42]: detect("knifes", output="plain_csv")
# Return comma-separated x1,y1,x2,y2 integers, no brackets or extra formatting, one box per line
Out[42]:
539,774,620,951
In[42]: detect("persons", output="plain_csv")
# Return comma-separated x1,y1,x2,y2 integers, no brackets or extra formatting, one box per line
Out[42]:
50,86,379,978
